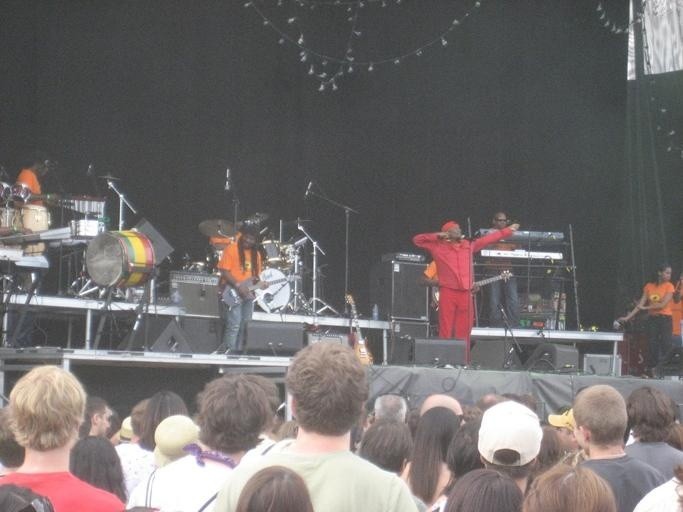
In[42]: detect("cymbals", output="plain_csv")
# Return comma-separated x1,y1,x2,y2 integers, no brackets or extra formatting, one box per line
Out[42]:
199,218,234,238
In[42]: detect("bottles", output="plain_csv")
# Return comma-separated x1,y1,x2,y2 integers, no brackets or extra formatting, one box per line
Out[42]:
372,303,378,321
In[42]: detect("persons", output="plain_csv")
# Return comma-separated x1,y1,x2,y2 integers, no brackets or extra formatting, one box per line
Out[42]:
481,211,519,328
673,272,683,337
412,220,519,364
217,226,269,354
616,263,676,379
1,338,682,511
8,152,52,347
415,259,480,337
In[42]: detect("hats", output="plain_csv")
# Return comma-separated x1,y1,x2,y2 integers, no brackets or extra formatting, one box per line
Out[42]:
117,414,137,444
477,400,544,468
549,408,580,432
439,220,462,233
151,415,200,468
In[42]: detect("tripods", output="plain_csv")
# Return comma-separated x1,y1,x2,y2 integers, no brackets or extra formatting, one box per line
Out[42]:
283,252,310,314
303,228,343,316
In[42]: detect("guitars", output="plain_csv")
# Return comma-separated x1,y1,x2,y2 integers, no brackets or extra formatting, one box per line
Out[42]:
346,294,373,366
221,274,302,307
430,269,514,310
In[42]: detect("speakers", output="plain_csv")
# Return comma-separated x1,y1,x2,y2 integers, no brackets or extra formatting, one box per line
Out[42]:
370,260,429,322
412,338,468,366
150,317,198,353
235,319,303,355
522,342,579,375
159,270,220,355
376,320,429,366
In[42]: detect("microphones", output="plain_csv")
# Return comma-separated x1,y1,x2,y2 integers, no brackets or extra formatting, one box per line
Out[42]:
223,168,231,193
460,234,475,242
301,180,314,201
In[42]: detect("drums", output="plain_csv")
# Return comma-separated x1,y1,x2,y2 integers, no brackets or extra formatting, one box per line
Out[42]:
19,205,50,232
85,230,154,289
281,244,301,265
257,269,291,313
260,240,284,263
0,182,10,206
10,183,32,202
0,206,17,232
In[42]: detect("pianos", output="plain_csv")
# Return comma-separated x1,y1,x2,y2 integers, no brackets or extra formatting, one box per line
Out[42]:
1,226,71,244
480,249,565,260
